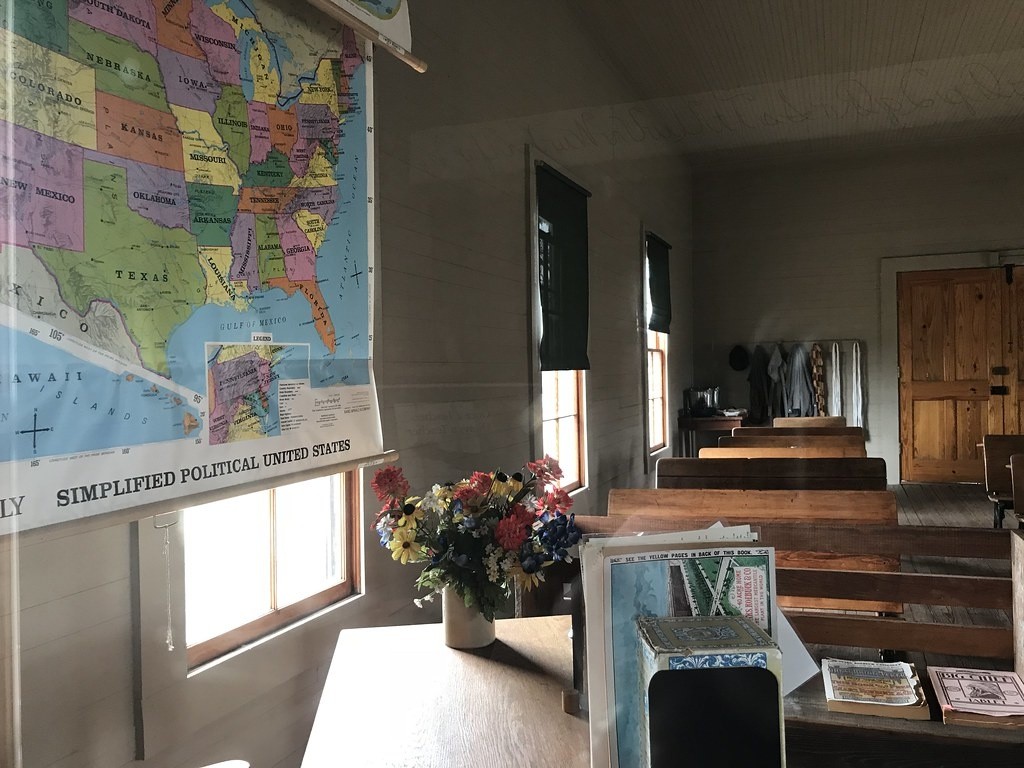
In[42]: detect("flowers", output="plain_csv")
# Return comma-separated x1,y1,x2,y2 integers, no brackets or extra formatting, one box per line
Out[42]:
367,454,582,574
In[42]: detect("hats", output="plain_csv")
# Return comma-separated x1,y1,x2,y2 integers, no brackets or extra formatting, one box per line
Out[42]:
729,344,749,370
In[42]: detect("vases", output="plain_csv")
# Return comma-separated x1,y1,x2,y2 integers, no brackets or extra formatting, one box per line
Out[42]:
442,574,496,649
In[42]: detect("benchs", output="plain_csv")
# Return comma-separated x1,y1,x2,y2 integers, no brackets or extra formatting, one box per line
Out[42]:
606,489,904,661
774,416,846,427
983,434,1024,528
732,427,863,436
1010,454,1024,529
697,448,869,457
655,457,888,489
718,436,866,447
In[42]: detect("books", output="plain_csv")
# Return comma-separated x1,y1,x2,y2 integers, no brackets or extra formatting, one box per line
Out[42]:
926,664,1024,732
820,657,934,720
600,542,782,768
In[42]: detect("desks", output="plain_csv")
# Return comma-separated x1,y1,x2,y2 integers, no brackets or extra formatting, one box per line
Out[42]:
298,612,590,768
678,407,747,459
